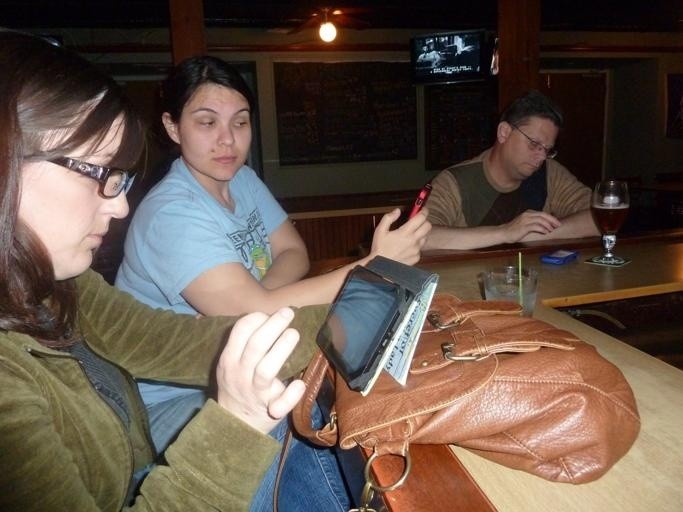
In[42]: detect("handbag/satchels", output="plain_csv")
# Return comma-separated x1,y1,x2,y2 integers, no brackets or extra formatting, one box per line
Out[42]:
289,290,643,488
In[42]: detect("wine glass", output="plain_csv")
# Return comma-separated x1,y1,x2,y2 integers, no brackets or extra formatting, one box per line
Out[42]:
590,179,629,265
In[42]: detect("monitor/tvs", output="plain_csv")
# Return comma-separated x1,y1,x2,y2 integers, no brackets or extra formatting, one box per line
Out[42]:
411,29,490,84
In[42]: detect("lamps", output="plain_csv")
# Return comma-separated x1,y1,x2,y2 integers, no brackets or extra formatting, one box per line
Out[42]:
319,8,336,43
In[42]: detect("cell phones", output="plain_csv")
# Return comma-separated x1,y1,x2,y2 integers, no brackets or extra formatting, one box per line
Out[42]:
405,186,431,224
540,247,578,266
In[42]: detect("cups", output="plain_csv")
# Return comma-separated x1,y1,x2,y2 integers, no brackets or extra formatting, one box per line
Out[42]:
483,266,539,320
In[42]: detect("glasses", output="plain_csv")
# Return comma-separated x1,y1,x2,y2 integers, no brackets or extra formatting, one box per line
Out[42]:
511,122,558,159
25,146,139,199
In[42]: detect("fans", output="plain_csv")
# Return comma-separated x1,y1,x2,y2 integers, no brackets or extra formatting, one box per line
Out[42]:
281,0,374,38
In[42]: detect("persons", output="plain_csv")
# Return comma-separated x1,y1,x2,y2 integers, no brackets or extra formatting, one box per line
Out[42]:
413,103,602,250
0,57,350,511
116,54,432,453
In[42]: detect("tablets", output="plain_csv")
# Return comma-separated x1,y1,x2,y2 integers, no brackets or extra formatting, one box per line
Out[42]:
314,267,410,393
